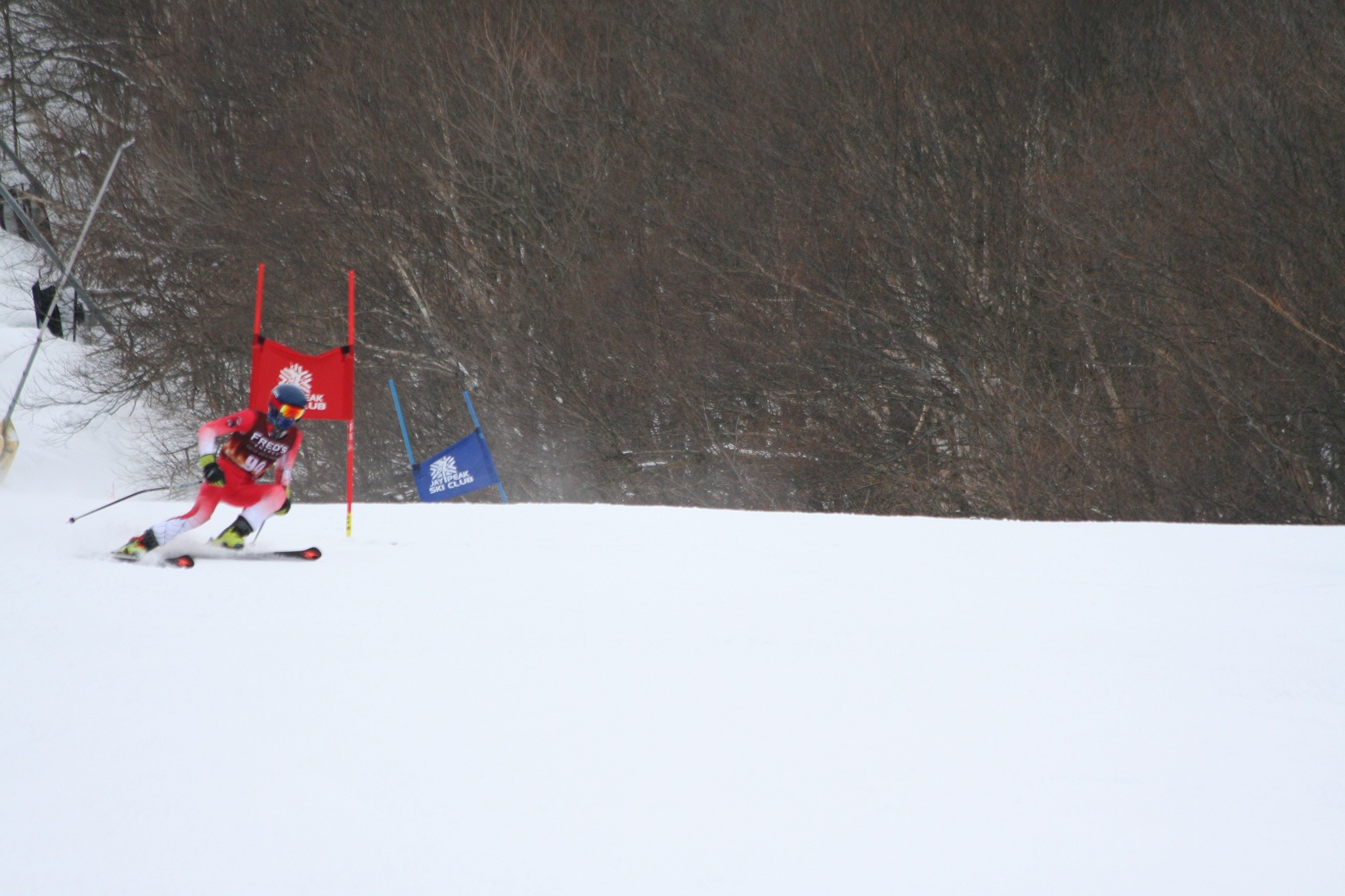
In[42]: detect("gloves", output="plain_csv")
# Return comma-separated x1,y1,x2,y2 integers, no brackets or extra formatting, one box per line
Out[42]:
198,454,226,487
277,486,294,515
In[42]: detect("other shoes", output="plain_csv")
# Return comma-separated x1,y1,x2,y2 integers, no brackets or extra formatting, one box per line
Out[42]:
114,527,159,562
213,515,253,548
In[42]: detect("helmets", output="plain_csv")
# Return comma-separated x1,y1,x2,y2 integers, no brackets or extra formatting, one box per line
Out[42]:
268,384,308,429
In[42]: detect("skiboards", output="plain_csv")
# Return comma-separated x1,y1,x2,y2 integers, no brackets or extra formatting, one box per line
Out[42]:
109,546,322,569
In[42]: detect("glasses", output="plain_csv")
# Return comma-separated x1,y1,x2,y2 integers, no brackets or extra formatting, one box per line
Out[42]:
270,393,305,421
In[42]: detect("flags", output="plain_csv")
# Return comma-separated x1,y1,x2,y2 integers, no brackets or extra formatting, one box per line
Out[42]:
250,334,354,421
412,427,498,503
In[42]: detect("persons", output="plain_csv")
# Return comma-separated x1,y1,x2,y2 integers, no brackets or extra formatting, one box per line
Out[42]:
112,384,308,560
